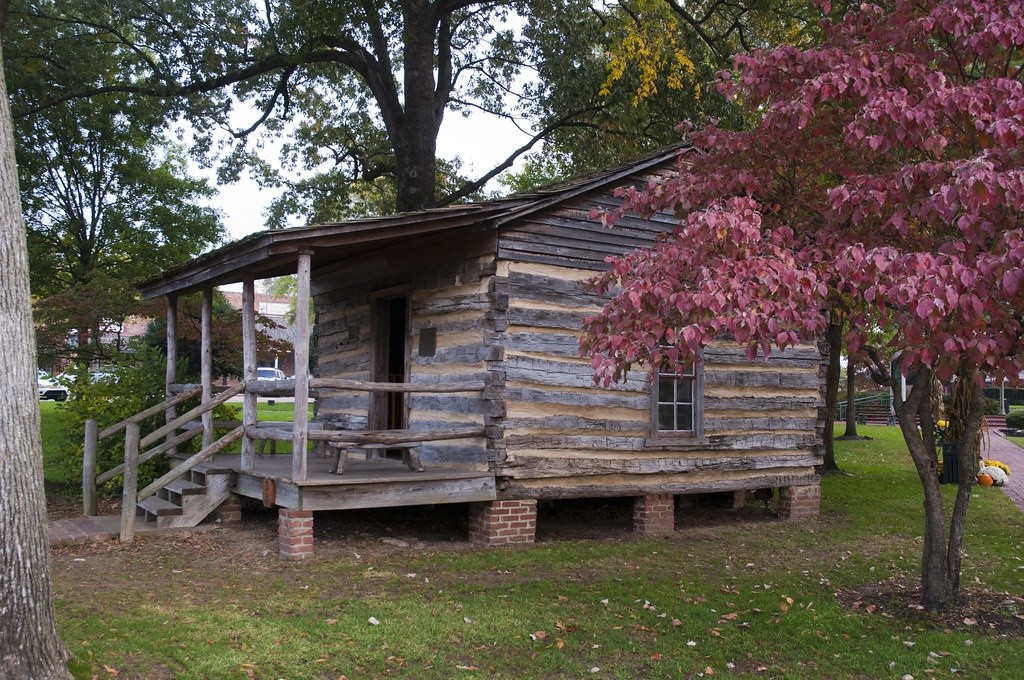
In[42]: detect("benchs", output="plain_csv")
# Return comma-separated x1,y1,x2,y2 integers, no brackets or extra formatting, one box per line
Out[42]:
329,439,424,475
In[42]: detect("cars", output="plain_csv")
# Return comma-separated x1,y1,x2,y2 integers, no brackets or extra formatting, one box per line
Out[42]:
56,365,137,386
287,374,315,379
37,368,69,402
256,367,288,380
